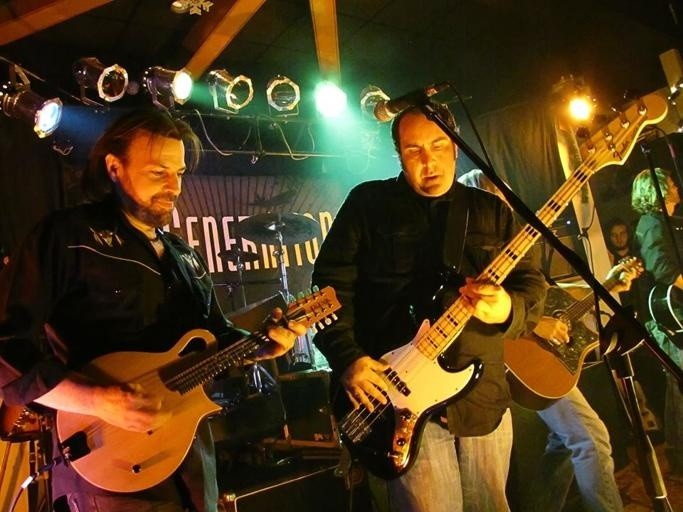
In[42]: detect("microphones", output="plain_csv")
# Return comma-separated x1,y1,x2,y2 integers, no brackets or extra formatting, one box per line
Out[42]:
374,80,449,122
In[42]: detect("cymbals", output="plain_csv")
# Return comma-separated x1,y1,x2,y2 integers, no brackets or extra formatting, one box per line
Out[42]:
218,249,261,263
237,213,321,246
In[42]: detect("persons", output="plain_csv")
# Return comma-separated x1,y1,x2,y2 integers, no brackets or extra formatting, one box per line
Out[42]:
630,167,682,484
459,168,625,511
1,107,308,511
605,215,667,448
311,101,548,512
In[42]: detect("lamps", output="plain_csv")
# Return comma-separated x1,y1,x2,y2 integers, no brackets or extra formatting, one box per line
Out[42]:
73,56,130,109
362,81,392,130
205,58,255,121
0,62,39,126
260,68,303,119
31,89,107,146
141,64,194,109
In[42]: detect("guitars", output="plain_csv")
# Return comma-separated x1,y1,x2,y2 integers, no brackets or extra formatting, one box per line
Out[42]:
648,284,683,348
329,89,669,483
503,255,643,411
